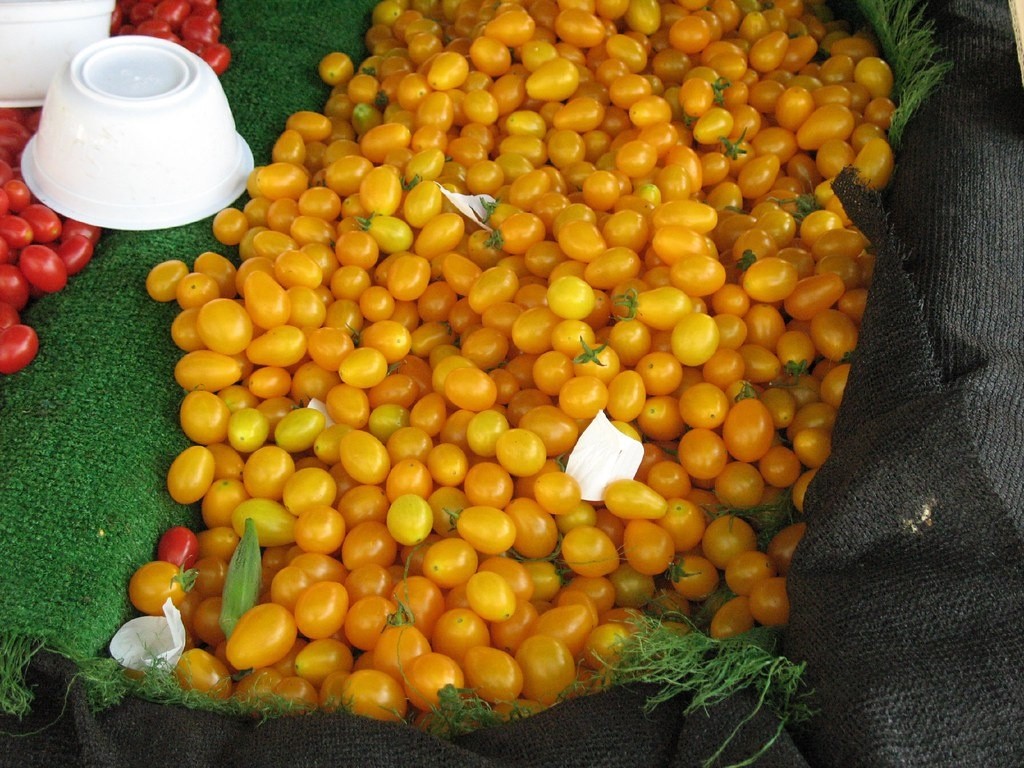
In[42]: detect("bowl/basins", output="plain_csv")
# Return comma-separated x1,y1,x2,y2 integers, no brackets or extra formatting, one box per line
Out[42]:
0,0,117,109
19,36,258,234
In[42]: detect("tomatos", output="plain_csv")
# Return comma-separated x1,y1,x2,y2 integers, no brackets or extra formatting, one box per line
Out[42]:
0,103,103,376
112,0,230,78
127,1,898,735
